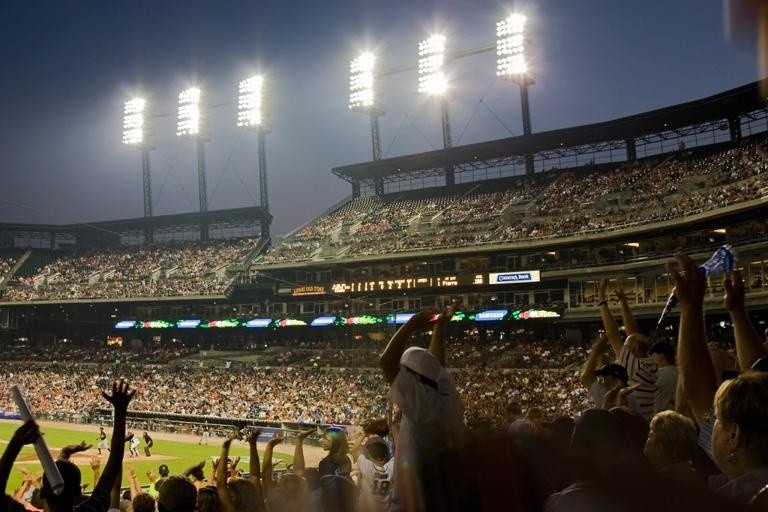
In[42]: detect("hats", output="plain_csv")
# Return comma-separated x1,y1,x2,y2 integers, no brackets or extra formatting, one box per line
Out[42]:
400,347,443,384
592,364,629,383
366,443,389,461
159,465,169,475
43,460,81,491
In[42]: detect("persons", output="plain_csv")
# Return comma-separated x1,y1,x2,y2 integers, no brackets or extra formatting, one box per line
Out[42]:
199,421,209,446
707,370,768,512
262,428,317,512
158,475,197,512
0,341,136,379
143,432,153,457
350,417,389,463
191,468,206,491
262,141,768,263
581,330,637,411
644,410,708,512
16,467,45,512
402,333,432,353
355,434,395,512
0,236,262,302
608,406,643,446
155,465,171,512
272,459,293,480
598,273,658,422
542,410,645,512
12,479,35,503
207,456,240,485
107,431,134,512
650,342,679,414
89,456,101,489
319,428,360,512
126,431,139,458
137,341,378,416
216,427,265,512
0,380,137,512
466,328,581,408
379,298,466,512
124,467,160,512
194,485,221,512
666,253,768,461
95,427,111,456
